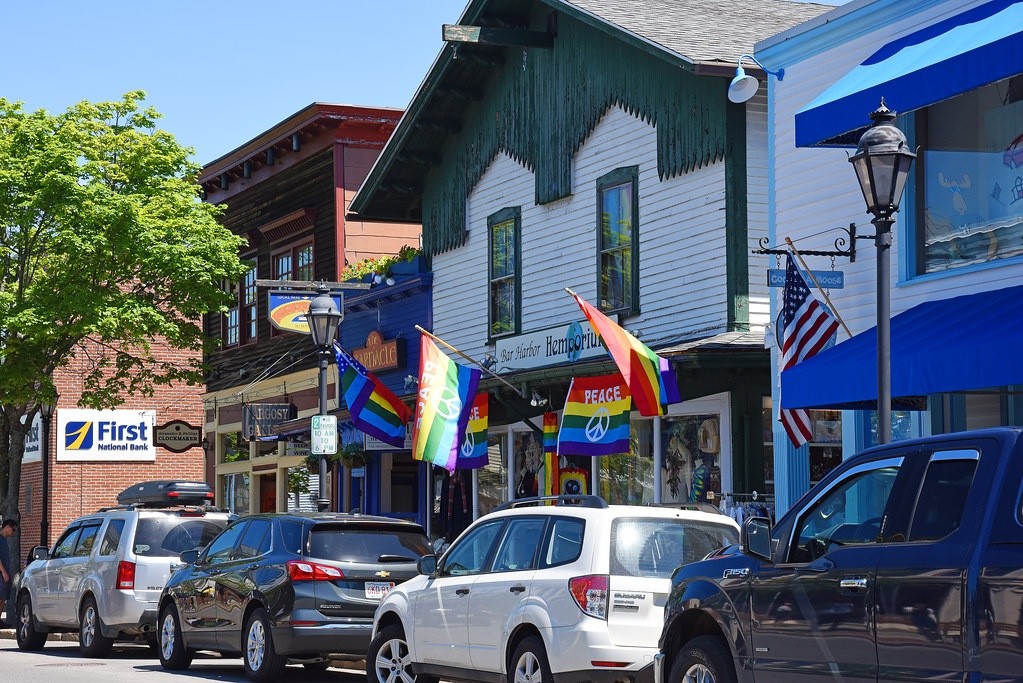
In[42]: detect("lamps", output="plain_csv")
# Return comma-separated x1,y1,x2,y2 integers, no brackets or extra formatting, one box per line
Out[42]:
201,188,207,200
203,437,214,458
293,135,301,152
530,388,548,407
222,176,227,190
267,150,275,166
244,162,251,178
728,55,784,103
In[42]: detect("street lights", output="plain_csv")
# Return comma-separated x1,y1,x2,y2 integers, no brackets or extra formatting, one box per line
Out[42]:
303,280,342,512
845,96,921,445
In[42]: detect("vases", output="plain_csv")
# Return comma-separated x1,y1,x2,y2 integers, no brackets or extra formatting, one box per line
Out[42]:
334,256,431,300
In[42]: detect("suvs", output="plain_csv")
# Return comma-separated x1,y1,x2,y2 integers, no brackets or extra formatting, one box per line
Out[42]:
155,512,435,682
13,479,237,656
653,427,1023,683
365,493,742,683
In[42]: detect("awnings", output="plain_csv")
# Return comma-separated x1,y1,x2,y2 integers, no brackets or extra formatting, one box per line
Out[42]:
781,285,1023,411
795,0,1023,148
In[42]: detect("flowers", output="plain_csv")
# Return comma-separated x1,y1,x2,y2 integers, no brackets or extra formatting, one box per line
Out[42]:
341,246,425,282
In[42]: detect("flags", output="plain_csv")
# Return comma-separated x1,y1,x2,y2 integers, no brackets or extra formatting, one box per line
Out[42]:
456,387,492,471
558,369,634,458
411,334,482,476
331,339,413,449
573,294,683,421
778,245,844,450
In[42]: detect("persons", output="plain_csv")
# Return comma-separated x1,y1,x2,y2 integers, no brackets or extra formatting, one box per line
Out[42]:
0,519,17,629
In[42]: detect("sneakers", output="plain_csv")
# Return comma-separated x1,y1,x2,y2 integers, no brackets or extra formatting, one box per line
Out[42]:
0,620,11,629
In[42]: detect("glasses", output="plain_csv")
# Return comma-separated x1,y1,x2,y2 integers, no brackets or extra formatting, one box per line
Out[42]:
9,526,15,532
